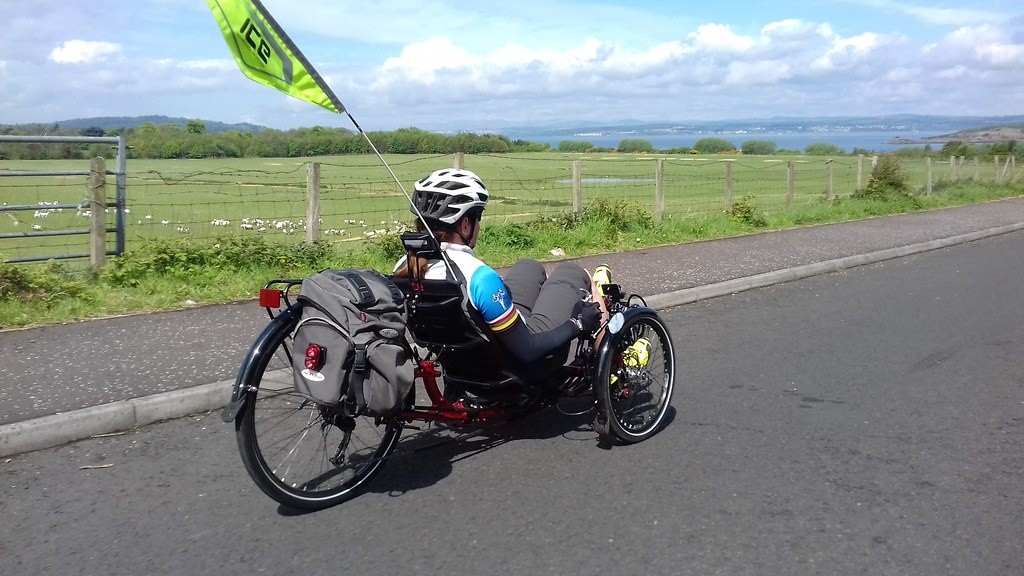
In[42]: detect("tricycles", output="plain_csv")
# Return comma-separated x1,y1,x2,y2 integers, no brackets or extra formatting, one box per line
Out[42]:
220,228,676,510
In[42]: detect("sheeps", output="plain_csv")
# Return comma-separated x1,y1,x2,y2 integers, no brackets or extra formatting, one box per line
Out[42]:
3,200,417,239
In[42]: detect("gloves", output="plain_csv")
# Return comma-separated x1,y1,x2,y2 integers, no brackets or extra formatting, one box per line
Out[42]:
570,299,603,333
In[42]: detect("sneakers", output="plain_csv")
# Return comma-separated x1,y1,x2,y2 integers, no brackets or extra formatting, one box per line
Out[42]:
610,337,652,384
592,263,612,301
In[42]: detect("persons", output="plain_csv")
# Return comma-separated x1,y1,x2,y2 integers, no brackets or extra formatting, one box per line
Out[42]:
394,169,652,398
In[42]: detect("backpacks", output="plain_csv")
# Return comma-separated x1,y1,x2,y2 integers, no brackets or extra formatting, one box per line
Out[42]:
290,268,415,418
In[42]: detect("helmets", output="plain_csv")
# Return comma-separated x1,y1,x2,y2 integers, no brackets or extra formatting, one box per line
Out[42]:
410,168,489,227
